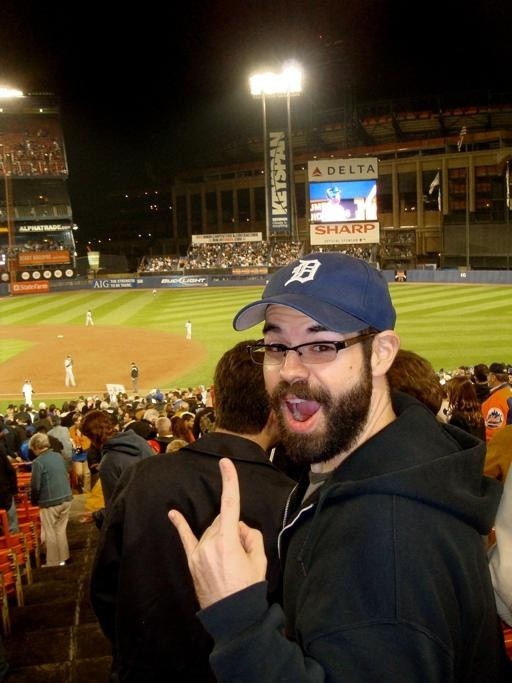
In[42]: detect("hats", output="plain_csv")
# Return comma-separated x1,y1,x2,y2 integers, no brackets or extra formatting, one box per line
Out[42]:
232,253,396,332
472,363,508,375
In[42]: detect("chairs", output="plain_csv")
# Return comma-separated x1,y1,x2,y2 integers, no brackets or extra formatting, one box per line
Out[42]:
0,449,74,636
500,622,512,657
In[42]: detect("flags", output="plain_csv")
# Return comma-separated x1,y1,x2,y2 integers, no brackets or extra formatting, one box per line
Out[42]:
429,173,440,194
456,126,467,150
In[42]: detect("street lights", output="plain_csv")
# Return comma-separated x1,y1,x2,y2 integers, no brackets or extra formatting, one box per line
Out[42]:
249,69,278,242
0,86,25,282
277,67,302,241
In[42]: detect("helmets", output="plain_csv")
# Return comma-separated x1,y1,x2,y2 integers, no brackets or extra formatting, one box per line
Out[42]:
325,186,341,199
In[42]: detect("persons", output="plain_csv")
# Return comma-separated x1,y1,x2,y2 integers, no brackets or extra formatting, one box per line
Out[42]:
321,186,346,221
1,232,512,683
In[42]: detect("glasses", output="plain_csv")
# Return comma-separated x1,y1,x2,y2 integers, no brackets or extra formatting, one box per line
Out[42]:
245,332,375,365
